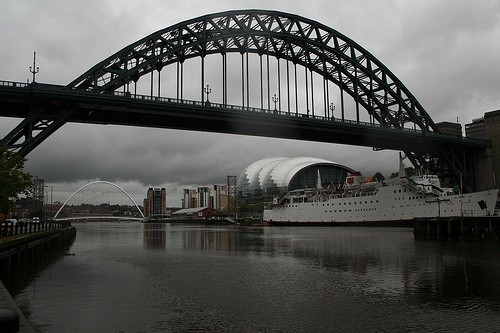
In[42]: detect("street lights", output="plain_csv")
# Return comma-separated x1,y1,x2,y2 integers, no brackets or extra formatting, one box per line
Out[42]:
29,51,40,83
330,102,336,121
271,93,279,113
204,84,211,105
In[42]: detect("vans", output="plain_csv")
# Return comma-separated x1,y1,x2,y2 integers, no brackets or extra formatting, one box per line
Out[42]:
30,216,40,223
2,218,17,227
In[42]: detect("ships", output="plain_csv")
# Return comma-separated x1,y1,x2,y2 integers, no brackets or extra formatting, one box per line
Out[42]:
260,151,500,226
